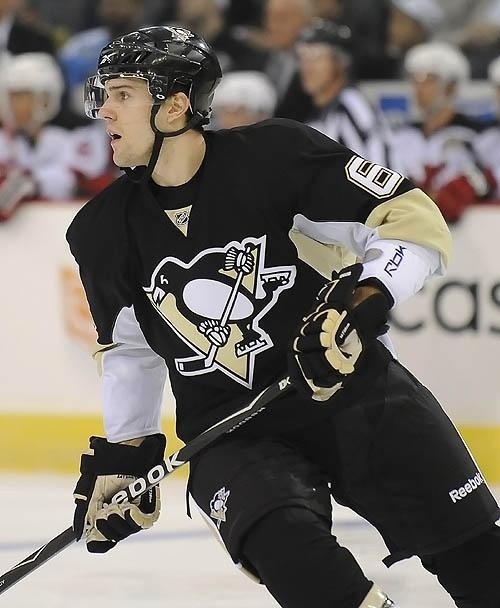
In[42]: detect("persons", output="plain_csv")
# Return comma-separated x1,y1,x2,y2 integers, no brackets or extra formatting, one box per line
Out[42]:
72,25,500,608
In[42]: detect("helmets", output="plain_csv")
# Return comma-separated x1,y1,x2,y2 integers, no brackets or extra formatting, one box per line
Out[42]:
295,15,369,64
96,24,223,124
211,72,276,109
404,44,468,80
0,52,64,122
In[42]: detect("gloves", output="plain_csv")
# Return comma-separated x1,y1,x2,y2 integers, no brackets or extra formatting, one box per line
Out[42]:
287,263,390,407
72,434,166,553
